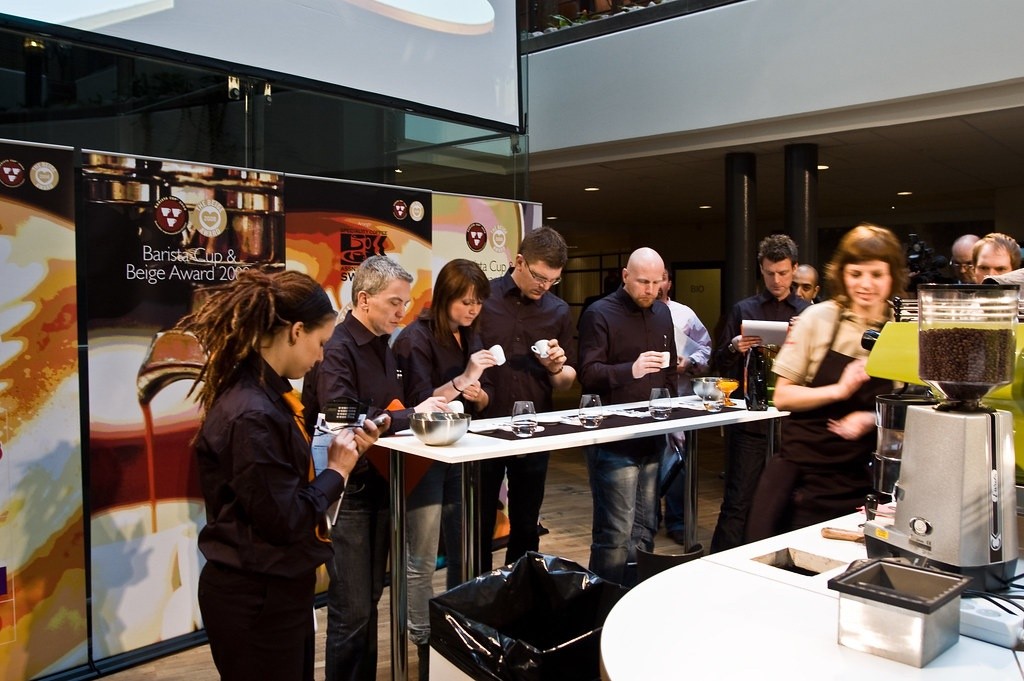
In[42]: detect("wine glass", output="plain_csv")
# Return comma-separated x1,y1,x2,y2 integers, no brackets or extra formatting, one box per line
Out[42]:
716,378,739,406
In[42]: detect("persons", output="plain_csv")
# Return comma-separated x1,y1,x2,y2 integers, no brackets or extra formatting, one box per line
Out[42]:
711,234,820,553
391,259,497,681
475,227,577,577
301,255,415,681
187,270,389,681
581,247,712,585
950,233,1021,285
747,224,914,544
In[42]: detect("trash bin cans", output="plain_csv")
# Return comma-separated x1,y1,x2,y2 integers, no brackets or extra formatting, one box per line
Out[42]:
429,552,629,681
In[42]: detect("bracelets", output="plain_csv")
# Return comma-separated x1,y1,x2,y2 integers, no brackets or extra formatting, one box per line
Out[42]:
547,366,563,376
728,343,736,353
451,379,463,392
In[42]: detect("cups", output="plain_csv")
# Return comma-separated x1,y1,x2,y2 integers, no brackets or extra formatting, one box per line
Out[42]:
659,351,670,368
579,394,603,429
531,339,552,358
488,344,505,366
702,378,724,411
511,400,537,437
649,387,672,420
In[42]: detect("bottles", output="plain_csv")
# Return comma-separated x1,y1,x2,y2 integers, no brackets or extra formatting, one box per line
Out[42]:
744,344,768,411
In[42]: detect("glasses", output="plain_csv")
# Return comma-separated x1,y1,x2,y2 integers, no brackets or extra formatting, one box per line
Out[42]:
949,256,974,270
525,258,562,285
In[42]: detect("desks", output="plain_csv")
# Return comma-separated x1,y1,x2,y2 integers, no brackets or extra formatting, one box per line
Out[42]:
599,500,1024,681
369,393,793,681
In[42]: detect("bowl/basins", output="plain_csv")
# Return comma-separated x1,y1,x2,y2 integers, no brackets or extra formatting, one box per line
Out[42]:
408,412,471,445
691,378,724,401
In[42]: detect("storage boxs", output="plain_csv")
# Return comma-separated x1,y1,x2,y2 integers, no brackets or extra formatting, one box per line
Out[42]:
826,557,974,670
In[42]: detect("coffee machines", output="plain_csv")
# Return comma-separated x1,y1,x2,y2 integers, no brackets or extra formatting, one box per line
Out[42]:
865,279,1019,596
